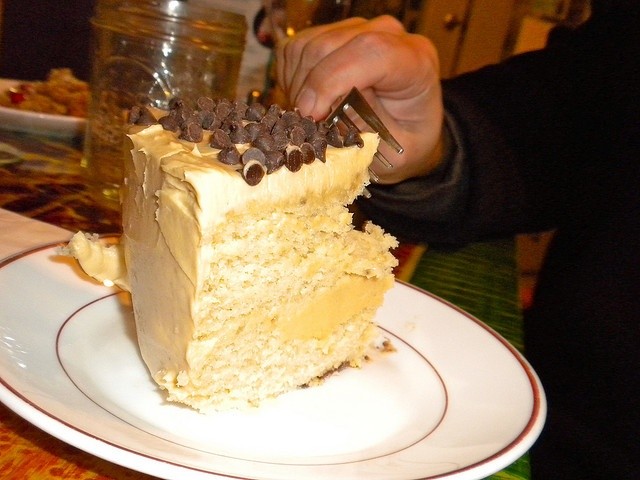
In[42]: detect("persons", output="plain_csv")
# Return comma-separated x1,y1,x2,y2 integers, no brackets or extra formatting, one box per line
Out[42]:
274,0,640,479
253,1,423,113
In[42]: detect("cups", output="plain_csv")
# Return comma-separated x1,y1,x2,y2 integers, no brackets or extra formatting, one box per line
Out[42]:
82,0,248,212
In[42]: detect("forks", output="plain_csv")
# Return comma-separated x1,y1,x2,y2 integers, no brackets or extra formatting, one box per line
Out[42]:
263,1,403,200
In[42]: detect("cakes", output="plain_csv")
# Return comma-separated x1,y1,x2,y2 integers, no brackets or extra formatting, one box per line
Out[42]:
61,95,401,418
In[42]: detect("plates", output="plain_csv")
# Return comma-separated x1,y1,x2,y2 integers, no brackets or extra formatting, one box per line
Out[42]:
0,77,88,139
0,232,550,479
